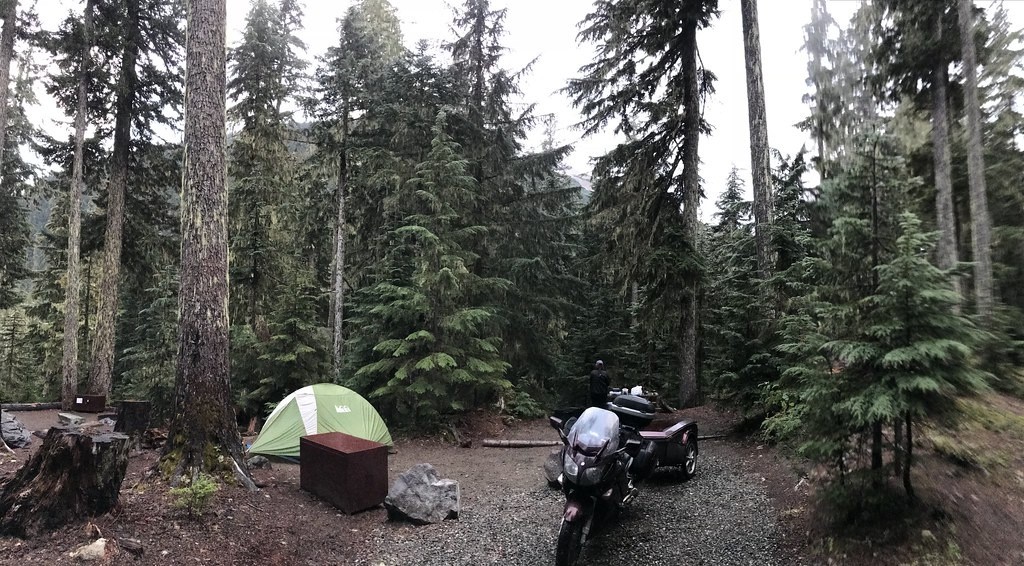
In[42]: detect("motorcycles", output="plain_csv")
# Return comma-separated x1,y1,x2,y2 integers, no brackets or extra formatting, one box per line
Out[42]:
547,395,658,566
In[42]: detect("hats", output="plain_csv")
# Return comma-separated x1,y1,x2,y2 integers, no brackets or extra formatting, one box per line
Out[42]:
595,360,603,367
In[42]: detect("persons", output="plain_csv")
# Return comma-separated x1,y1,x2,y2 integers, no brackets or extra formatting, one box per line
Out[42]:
590,360,611,410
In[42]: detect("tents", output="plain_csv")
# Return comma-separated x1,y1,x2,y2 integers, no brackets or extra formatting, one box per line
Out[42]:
245,383,397,465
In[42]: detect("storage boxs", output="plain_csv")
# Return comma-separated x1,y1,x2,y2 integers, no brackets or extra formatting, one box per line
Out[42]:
301,431,390,515
73,394,106,412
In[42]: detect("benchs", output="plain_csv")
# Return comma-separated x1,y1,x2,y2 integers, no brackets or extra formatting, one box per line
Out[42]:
58,413,84,426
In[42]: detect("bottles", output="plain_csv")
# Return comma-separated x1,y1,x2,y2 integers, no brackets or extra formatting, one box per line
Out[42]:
245,444,252,448
